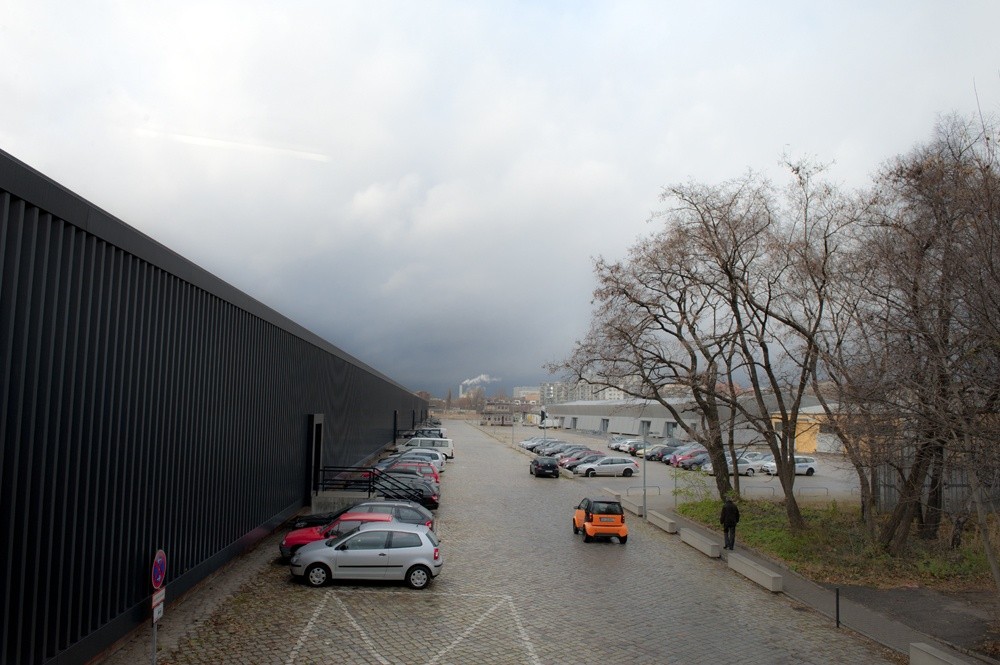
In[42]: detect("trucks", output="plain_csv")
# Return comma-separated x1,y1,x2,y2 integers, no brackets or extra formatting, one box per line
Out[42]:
538,418,559,429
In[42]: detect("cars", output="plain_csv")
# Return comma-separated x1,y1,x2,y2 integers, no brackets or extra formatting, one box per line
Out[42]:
762,456,818,476
279,512,408,559
519,436,606,470
290,522,444,590
574,457,639,477
607,434,774,470
701,458,760,477
529,455,559,478
572,496,628,545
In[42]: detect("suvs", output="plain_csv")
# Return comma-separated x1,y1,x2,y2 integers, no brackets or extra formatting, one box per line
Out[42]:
393,437,455,460
404,429,443,438
343,447,445,507
292,500,438,534
